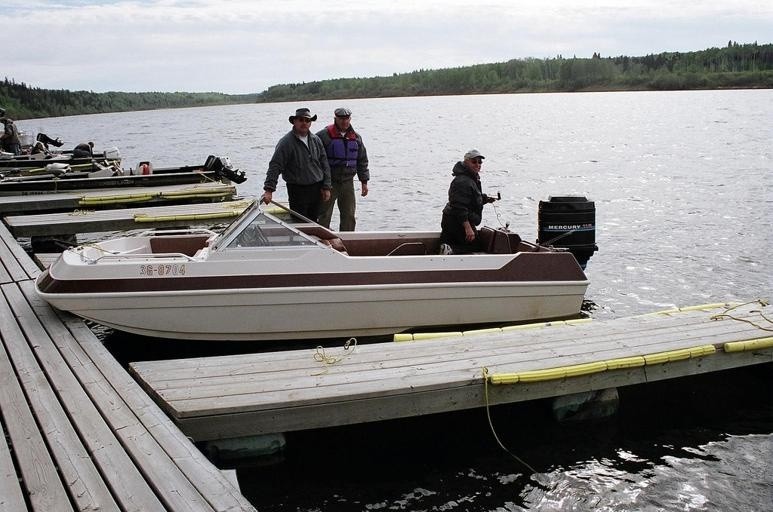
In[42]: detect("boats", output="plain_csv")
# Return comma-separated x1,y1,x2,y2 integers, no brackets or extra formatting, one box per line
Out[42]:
33,195,598,342
0,130,248,197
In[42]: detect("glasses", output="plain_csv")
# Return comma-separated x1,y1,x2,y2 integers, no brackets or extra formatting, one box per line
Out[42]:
299,118,310,122
471,160,483,164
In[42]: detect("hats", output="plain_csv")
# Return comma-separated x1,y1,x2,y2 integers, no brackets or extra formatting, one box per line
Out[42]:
463,149,485,161
334,107,352,117
289,108,317,125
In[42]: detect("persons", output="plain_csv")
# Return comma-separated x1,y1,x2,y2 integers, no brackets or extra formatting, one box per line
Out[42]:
261,107,369,231
439,149,494,254
0,119,20,155
74,142,94,157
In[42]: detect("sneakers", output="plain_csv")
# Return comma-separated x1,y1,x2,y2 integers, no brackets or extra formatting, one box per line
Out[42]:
439,243,454,255
